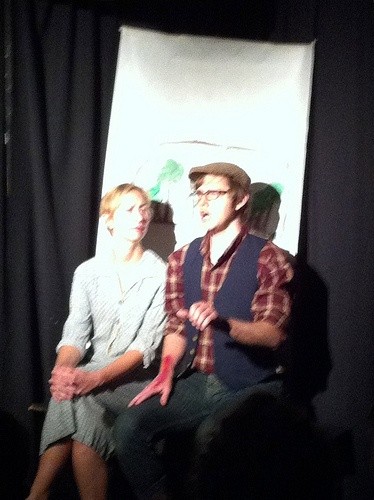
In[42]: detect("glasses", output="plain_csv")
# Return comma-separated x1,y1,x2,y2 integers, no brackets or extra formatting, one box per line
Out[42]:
189,191,228,200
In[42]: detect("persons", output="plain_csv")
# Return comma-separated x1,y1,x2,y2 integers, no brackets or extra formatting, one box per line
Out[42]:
112,162,295,500
22,183,172,500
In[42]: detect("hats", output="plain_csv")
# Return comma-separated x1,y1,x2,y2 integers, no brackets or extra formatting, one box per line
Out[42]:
189,163,250,191
